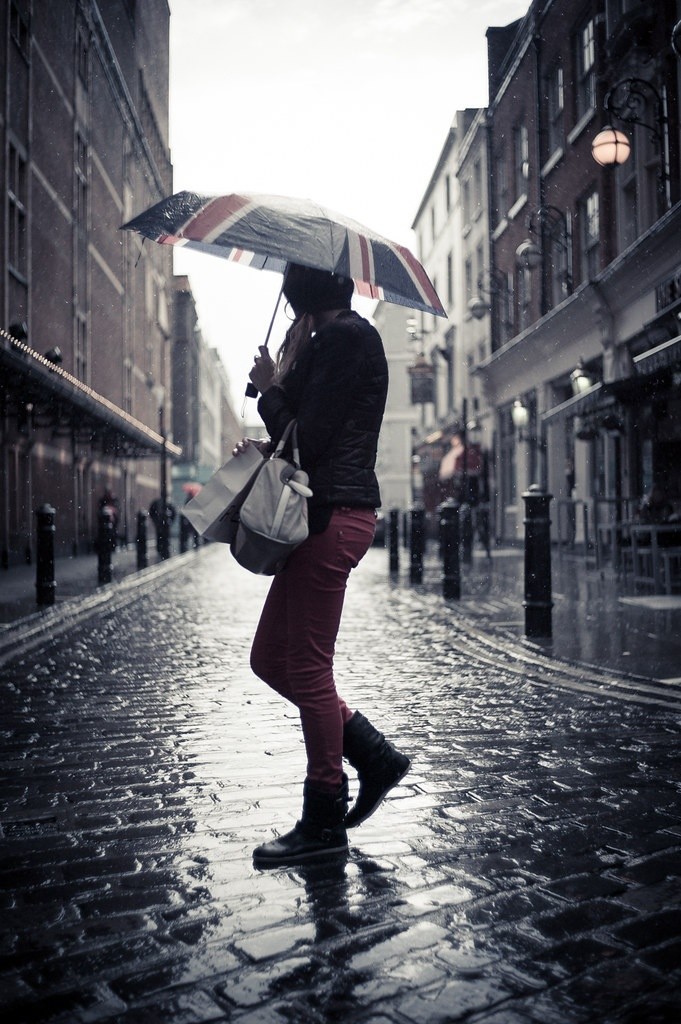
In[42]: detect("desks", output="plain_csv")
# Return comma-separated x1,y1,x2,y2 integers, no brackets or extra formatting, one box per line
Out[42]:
630,524,681,595
597,524,629,584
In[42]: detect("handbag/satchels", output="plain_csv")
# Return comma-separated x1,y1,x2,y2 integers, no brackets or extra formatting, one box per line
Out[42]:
181,441,270,544
230,419,314,576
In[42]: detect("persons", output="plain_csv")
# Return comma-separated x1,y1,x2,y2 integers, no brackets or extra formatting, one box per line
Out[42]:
151,497,176,550
459,503,472,561
474,502,492,558
232,262,411,863
98,484,121,551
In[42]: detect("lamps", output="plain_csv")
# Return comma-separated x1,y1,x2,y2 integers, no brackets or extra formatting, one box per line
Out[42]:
46,345,64,371
10,319,29,352
516,203,572,294
591,75,680,194
468,264,511,339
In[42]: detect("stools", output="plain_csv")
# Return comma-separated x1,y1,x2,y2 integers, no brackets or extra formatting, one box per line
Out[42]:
611,539,681,594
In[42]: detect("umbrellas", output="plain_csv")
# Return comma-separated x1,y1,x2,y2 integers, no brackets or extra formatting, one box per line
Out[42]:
118,190,448,400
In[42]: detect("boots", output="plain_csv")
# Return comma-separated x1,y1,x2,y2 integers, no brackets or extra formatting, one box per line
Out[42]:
340,708,413,829
252,771,354,863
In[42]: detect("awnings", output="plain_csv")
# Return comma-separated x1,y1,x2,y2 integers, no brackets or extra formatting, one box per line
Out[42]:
540,335,681,426
